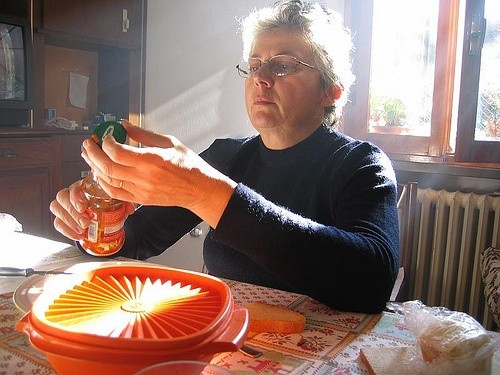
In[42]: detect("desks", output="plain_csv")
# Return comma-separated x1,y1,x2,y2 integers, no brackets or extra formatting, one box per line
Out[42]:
0,232,500,375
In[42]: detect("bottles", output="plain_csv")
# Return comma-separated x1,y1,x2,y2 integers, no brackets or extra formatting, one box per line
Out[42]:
74,121,127,260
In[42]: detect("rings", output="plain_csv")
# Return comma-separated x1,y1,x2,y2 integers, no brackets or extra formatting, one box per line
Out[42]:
119,180,123,190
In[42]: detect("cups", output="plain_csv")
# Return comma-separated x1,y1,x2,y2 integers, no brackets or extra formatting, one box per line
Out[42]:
134,360,234,375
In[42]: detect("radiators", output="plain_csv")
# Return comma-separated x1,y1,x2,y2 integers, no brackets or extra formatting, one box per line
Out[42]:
409,186,500,334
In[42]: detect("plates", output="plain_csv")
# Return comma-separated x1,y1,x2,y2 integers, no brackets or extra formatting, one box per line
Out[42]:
13,259,140,314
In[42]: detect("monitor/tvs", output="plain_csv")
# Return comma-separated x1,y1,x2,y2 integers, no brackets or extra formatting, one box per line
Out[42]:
0,14,36,127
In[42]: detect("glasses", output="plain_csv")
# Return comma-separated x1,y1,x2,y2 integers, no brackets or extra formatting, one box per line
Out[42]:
236,55,321,78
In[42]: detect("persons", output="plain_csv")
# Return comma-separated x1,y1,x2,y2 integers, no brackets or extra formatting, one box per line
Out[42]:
49,0,400,316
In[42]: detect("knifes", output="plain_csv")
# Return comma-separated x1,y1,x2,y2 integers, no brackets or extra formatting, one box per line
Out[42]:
0,267,74,277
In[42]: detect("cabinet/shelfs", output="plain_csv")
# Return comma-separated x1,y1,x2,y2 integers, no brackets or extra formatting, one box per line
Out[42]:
0,1,149,244
